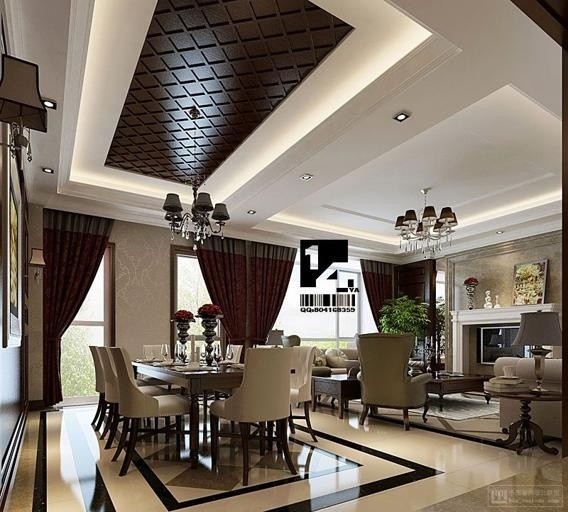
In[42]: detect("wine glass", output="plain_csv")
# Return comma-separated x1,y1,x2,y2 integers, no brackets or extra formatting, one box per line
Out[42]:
158,342,223,375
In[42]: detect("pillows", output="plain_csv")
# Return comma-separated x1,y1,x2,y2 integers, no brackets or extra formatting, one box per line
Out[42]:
325,347,348,368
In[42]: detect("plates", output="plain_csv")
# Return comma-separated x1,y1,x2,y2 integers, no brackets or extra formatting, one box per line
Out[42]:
232,364,244,369
448,374,464,377
500,377,518,379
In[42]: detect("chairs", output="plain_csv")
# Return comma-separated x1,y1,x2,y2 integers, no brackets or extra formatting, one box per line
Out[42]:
88,338,318,486
353,332,433,431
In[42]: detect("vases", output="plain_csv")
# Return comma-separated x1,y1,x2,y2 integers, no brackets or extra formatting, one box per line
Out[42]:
194,314,224,367
465,284,476,310
171,318,192,363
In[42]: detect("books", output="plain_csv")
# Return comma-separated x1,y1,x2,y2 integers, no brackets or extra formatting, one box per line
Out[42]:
483,374,531,394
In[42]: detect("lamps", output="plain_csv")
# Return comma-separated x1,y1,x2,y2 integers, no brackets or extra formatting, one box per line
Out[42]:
0,52,50,163
394,187,459,260
161,106,230,254
23,247,47,287
510,308,562,396
265,328,284,347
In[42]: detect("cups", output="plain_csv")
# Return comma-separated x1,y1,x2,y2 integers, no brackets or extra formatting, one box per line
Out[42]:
453,368,459,376
219,365,225,372
503,366,516,377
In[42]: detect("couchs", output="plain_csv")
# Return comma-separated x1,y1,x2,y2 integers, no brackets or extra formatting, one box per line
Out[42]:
494,355,562,440
312,347,358,375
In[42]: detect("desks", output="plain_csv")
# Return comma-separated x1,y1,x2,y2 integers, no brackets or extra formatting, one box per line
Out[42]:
311,373,361,420
425,373,497,412
482,381,562,456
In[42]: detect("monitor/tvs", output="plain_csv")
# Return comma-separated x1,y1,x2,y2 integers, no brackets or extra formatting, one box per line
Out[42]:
480,326,530,365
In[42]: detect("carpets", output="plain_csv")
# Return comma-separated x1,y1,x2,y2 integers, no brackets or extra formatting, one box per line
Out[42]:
351,392,500,422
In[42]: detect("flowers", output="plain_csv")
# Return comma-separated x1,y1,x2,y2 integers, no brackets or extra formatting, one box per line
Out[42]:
463,276,480,285
174,307,193,319
197,303,224,314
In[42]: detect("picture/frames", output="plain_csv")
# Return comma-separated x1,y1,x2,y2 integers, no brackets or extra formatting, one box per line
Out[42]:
510,258,549,305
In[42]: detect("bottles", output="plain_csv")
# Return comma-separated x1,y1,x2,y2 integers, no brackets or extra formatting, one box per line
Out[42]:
493,295,499,308
